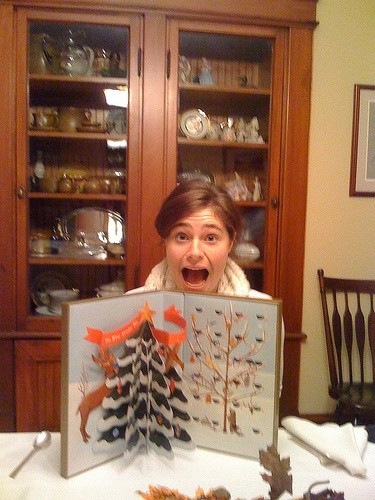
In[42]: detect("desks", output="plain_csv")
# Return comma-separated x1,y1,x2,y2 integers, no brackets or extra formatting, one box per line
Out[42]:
0,434,375,500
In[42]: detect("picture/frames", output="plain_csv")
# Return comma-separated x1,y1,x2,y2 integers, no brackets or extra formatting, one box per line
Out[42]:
349,83,375,197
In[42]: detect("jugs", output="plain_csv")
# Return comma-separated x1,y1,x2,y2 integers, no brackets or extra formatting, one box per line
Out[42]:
58,105,91,134
92,48,120,77
59,29,95,76
30,32,59,75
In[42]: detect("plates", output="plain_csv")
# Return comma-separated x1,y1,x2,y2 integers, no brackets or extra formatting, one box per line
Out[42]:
31,272,70,307
35,305,59,316
178,108,210,140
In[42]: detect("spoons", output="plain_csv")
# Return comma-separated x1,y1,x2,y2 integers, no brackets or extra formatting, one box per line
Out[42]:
9,430,52,477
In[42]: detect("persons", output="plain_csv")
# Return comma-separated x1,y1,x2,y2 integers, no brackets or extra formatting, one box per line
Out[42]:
124,180,284,398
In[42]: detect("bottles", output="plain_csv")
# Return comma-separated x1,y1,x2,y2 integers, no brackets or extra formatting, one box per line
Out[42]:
34,150,45,178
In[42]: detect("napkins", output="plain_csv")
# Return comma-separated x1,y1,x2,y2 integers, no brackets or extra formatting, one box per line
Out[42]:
281,416,368,476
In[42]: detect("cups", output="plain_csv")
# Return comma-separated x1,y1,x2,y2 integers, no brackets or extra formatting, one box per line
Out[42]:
95,281,124,298
31,112,59,127
40,175,126,194
47,288,79,314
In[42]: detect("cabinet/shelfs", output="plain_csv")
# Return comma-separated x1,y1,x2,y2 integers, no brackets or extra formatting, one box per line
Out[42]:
0,0,319,434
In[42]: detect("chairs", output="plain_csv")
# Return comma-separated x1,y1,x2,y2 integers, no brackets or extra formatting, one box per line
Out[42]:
317,267,375,442
